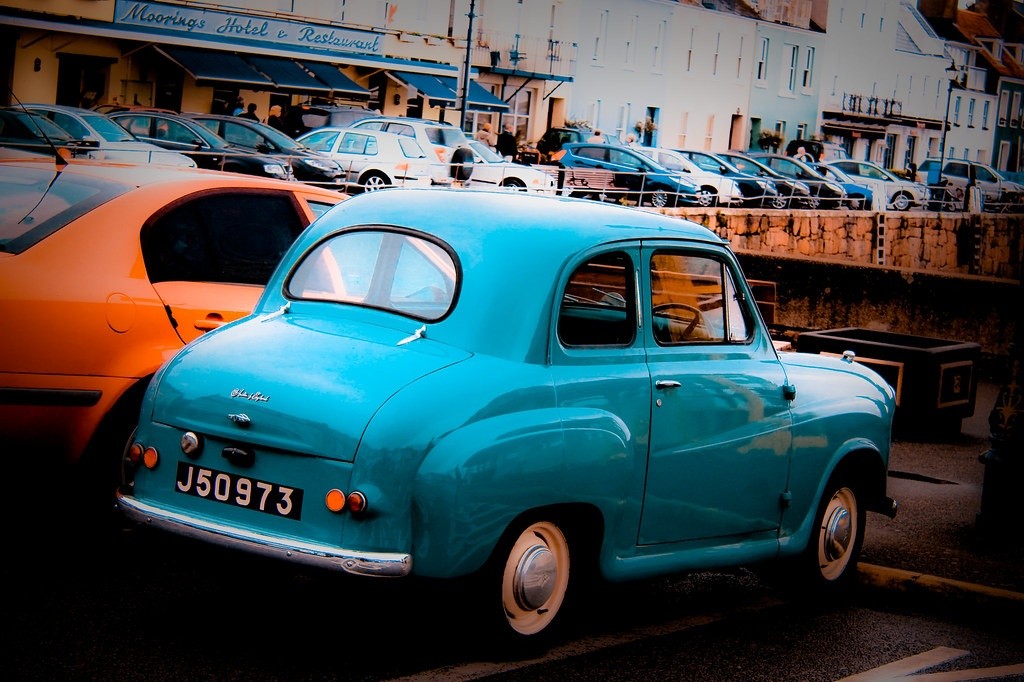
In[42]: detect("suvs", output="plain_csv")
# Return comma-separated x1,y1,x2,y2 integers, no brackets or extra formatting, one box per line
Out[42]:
283,104,475,188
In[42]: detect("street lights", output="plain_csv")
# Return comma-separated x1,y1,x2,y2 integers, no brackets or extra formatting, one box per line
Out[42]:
938,59,960,186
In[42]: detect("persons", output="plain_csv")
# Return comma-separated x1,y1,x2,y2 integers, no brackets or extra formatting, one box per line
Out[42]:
793,146,807,164
495,123,519,158
587,128,605,144
216,96,262,121
624,133,636,149
473,122,493,148
267,104,284,131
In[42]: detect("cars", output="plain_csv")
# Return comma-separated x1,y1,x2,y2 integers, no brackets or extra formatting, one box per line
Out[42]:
751,154,848,209
13,102,197,169
180,112,347,191
613,146,744,205
672,149,777,204
537,127,624,156
815,159,930,211
0,107,112,162
294,126,433,194
717,152,811,209
558,142,702,208
90,103,302,182
804,162,890,211
916,157,1024,214
468,140,556,194
778,139,859,174
117,186,897,651
1,144,456,519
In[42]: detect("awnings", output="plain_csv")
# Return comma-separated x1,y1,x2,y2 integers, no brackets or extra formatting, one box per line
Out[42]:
479,63,577,86
151,42,372,101
385,68,512,114
